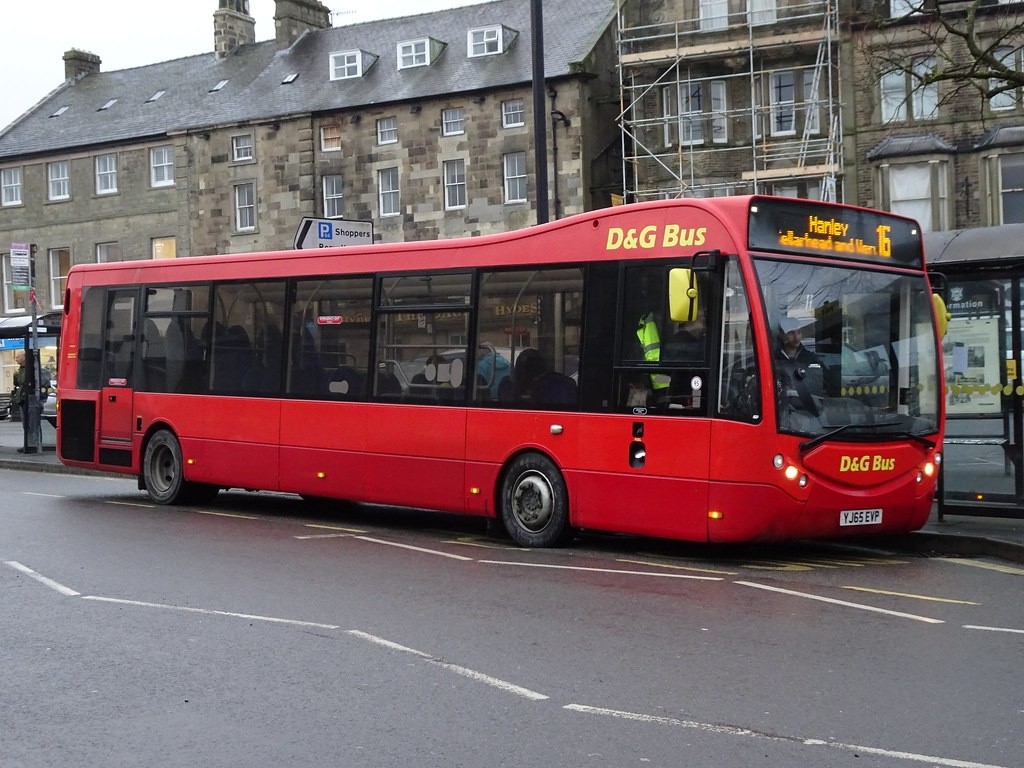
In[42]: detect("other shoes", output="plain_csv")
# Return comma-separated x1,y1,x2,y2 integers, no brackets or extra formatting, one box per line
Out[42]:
17,447,25,453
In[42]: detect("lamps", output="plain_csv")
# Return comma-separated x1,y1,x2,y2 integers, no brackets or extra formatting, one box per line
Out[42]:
350,115,360,124
473,96,485,104
410,106,421,114
198,134,211,139
550,110,572,128
268,124,280,130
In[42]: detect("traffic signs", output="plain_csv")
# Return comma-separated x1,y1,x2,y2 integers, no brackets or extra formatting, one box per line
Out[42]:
293,216,375,251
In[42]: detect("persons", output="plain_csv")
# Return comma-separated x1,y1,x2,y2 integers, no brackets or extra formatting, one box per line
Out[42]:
772,317,824,394
215,318,551,411
13,348,44,454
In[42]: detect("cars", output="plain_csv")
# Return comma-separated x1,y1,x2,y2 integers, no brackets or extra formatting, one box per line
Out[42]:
721,339,892,420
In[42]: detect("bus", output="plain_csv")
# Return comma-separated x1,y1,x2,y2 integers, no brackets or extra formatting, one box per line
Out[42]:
58,194,954,547
